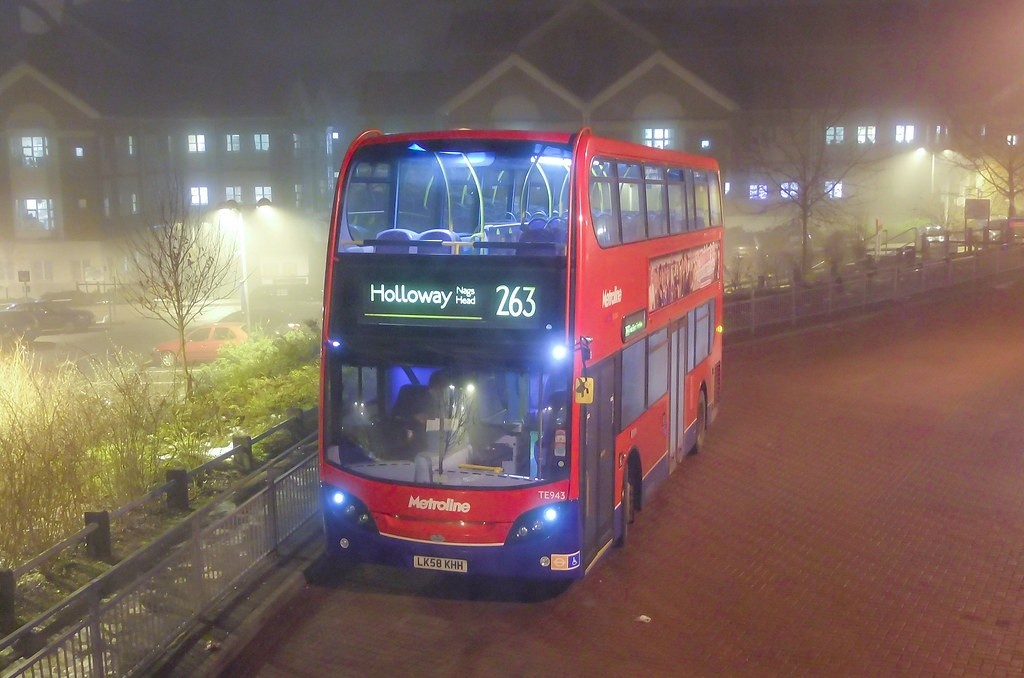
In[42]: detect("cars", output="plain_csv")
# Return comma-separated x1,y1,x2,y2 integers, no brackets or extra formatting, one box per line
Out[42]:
0,298,98,348
726,230,760,262
826,231,865,259
782,231,812,258
981,215,1008,241
149,324,256,368
925,226,947,245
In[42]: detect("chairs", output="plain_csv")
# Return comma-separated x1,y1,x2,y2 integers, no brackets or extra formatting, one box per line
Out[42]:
380,383,428,461
374,208,715,256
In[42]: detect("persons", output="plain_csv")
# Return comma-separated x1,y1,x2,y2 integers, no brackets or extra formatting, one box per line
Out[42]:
407,370,462,454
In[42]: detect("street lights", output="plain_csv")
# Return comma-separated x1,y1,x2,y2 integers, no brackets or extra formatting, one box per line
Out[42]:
218,197,274,339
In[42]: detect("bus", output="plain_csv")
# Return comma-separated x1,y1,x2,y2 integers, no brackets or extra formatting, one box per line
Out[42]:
315,128,727,582
